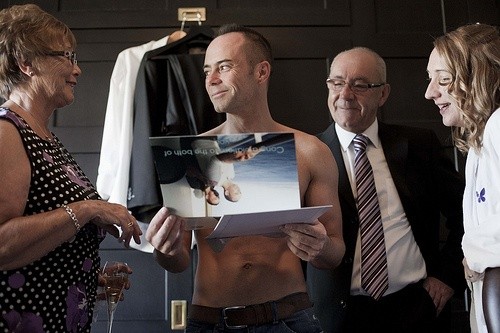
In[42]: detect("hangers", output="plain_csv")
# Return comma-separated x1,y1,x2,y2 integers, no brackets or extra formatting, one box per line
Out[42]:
166,8,215,55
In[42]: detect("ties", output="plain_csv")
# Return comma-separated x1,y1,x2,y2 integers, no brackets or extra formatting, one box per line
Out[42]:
351,133,389,301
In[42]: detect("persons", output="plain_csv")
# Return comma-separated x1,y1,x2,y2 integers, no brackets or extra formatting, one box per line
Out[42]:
144,22,346,333
178,136,259,206
424,23,500,333
0,4,143,333
298,45,472,333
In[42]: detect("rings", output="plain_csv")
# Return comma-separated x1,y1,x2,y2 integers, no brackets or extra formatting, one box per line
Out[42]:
127,221,134,228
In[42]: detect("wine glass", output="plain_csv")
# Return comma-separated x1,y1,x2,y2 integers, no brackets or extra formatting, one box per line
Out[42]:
102,260,128,333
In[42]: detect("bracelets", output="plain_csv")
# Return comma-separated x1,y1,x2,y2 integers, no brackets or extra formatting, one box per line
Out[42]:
60,204,81,235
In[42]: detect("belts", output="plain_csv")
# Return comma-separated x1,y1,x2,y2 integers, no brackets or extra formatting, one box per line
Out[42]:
188,292,311,330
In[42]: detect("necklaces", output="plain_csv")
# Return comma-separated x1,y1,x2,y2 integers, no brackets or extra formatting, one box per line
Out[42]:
9,98,72,163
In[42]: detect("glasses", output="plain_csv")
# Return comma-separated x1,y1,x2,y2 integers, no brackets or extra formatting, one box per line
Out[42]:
325,78,386,92
40,51,77,65
